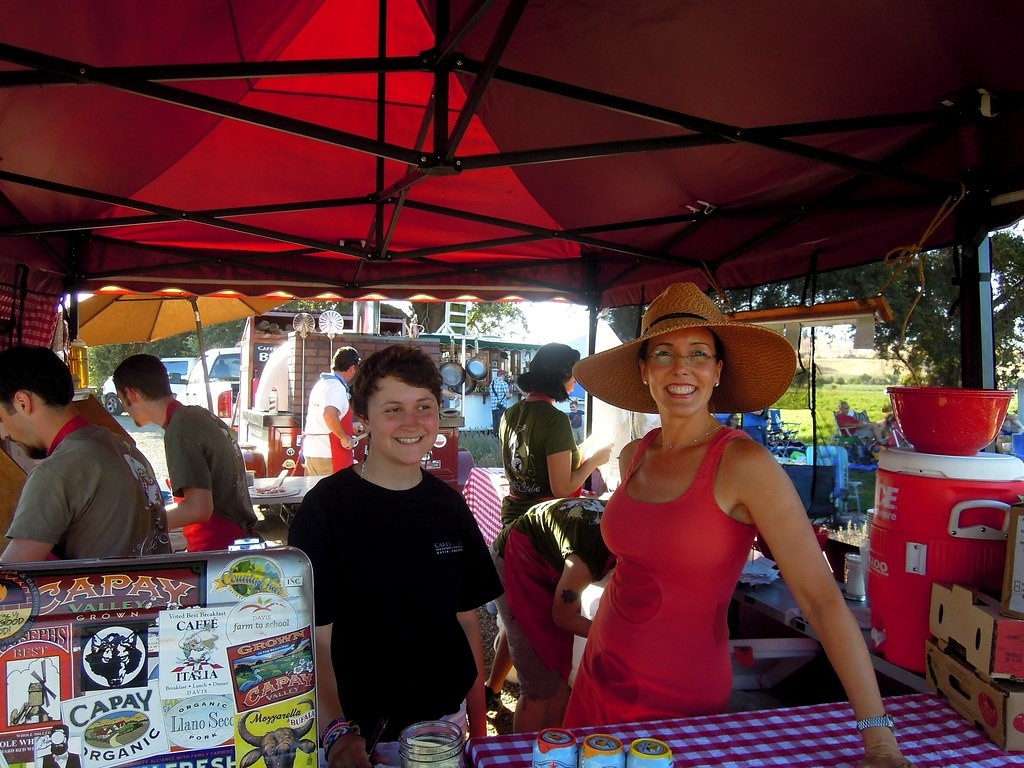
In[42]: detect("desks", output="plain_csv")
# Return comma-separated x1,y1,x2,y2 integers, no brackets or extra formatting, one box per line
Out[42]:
247,475,330,528
458,692,1024,768
730,578,938,696
462,467,511,547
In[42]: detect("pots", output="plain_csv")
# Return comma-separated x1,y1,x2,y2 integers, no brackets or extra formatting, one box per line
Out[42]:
438,336,488,395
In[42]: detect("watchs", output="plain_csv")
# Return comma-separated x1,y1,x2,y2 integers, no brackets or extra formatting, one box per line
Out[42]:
857,714,895,733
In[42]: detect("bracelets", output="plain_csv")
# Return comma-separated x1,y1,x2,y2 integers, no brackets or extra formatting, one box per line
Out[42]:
321,718,360,763
341,436,350,448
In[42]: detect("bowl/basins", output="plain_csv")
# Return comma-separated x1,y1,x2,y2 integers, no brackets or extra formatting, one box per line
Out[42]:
886,385,1017,457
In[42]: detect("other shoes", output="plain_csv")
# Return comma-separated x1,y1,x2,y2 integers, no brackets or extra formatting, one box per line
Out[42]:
482,683,506,713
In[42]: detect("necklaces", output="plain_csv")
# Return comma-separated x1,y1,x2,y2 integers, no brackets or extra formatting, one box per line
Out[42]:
661,425,726,448
361,460,366,479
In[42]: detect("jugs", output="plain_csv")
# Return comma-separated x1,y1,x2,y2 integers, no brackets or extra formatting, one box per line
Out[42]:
404,316,425,339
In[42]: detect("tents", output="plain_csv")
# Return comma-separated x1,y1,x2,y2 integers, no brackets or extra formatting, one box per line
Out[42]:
0,0,1024,768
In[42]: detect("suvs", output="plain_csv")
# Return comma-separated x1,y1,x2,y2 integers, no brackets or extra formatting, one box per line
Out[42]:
100,357,198,416
186,347,242,431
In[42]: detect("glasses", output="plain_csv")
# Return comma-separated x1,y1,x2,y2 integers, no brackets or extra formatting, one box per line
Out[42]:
645,350,718,367
844,406,850,410
734,417,740,421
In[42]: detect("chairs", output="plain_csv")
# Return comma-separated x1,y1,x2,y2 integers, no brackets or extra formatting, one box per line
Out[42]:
715,408,801,459
829,409,876,443
243,452,267,478
784,463,838,526
807,445,862,514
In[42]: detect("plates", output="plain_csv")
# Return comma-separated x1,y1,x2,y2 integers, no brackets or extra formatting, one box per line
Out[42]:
247,486,301,498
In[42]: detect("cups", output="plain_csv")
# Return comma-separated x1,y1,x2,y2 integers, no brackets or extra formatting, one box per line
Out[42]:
844,554,867,602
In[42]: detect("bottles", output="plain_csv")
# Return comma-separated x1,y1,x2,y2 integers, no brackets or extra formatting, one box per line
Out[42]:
398,721,463,768
269,386,278,415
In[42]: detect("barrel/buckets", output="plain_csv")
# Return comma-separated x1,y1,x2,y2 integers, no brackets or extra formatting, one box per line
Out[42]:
864,445,1023,673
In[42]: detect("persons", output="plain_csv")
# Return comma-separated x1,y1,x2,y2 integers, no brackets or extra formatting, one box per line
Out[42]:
835,401,884,443
488,497,617,734
0,344,172,563
727,414,739,428
483,342,615,715
303,346,361,476
562,282,913,768
568,401,584,443
488,369,512,440
113,354,258,552
286,345,505,768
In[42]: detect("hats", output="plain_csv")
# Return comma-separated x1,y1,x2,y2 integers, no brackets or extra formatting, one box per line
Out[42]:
497,370,508,375
573,282,796,414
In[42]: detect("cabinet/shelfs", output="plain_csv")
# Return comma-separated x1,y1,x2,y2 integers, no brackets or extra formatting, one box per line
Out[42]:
352,416,465,493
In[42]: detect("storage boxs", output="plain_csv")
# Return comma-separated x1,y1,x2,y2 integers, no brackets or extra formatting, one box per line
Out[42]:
1000,495,1024,621
924,639,1024,754
245,470,256,487
729,638,821,714
928,582,1024,684
